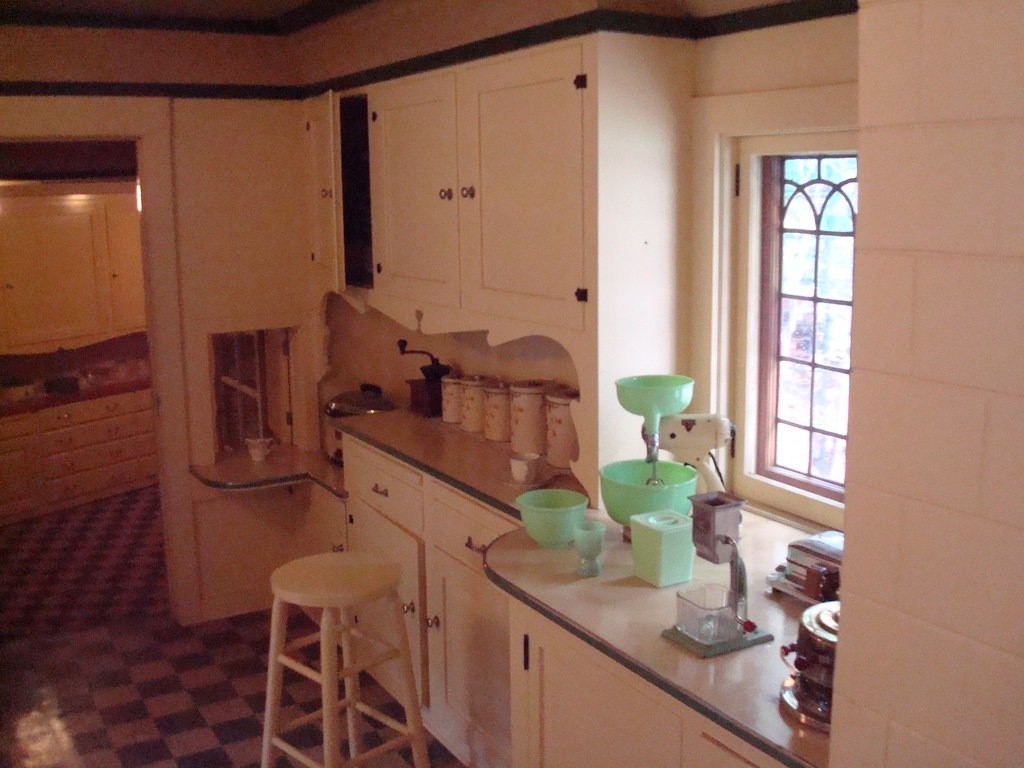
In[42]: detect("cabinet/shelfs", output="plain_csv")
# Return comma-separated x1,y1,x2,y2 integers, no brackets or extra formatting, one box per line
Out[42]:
329,34,828,768
0,380,162,527
0,179,149,355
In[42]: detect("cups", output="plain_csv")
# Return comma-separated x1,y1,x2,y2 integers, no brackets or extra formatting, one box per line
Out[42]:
510,453,540,482
246,437,273,462
572,521,606,577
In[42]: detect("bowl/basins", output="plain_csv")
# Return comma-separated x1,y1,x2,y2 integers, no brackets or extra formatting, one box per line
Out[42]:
515,488,590,548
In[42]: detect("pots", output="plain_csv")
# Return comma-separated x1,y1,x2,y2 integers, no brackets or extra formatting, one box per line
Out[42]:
323,382,395,468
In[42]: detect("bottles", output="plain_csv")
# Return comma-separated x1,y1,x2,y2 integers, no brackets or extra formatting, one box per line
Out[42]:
440,374,462,422
457,373,487,432
543,387,578,468
482,380,512,442
508,379,547,456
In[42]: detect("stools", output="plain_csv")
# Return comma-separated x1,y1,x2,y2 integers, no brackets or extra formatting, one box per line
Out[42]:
259,550,433,768
